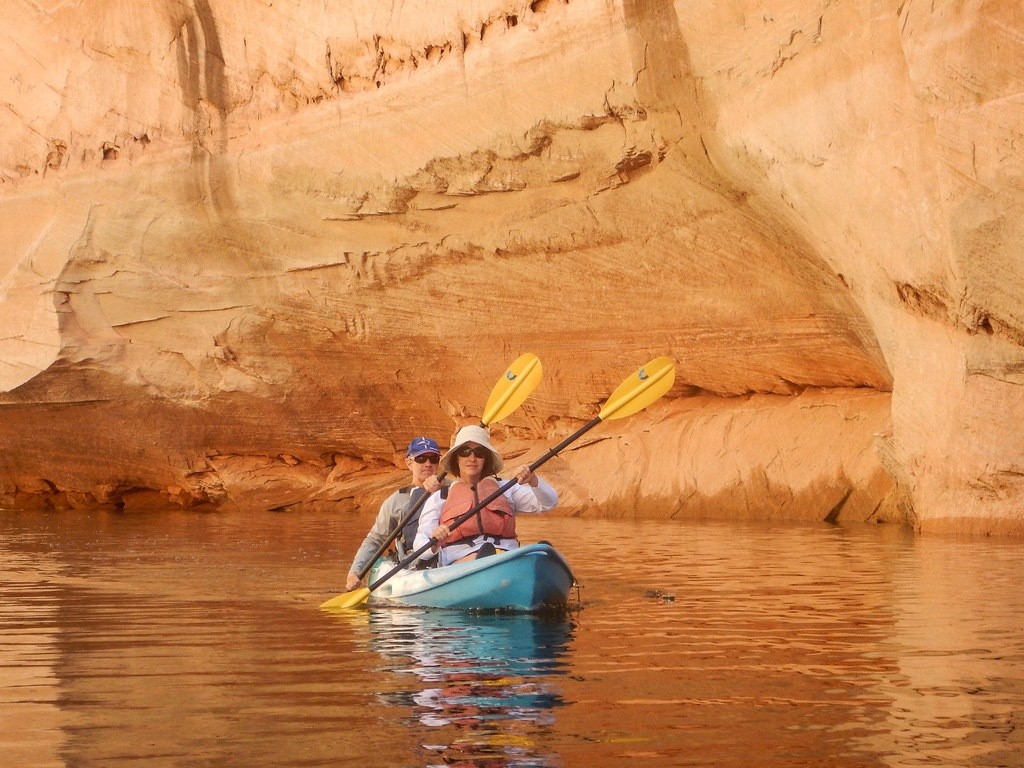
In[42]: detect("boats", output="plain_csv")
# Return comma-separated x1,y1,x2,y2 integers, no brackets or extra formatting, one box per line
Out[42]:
367,542,578,617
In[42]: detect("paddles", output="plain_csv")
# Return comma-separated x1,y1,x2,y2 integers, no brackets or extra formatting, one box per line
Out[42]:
350,352,546,592
317,355,677,616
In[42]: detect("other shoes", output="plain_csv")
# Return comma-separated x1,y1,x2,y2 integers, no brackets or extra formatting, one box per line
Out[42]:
538,540,553,548
476,542,497,560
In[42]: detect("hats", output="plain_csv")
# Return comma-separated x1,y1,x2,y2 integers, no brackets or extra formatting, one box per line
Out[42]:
406,436,442,460
440,425,504,480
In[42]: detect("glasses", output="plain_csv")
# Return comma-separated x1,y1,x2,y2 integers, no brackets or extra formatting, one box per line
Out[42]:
456,445,489,458
409,455,442,464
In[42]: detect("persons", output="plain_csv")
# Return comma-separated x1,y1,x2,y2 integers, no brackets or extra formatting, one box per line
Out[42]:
345,436,454,591
412,425,559,567
